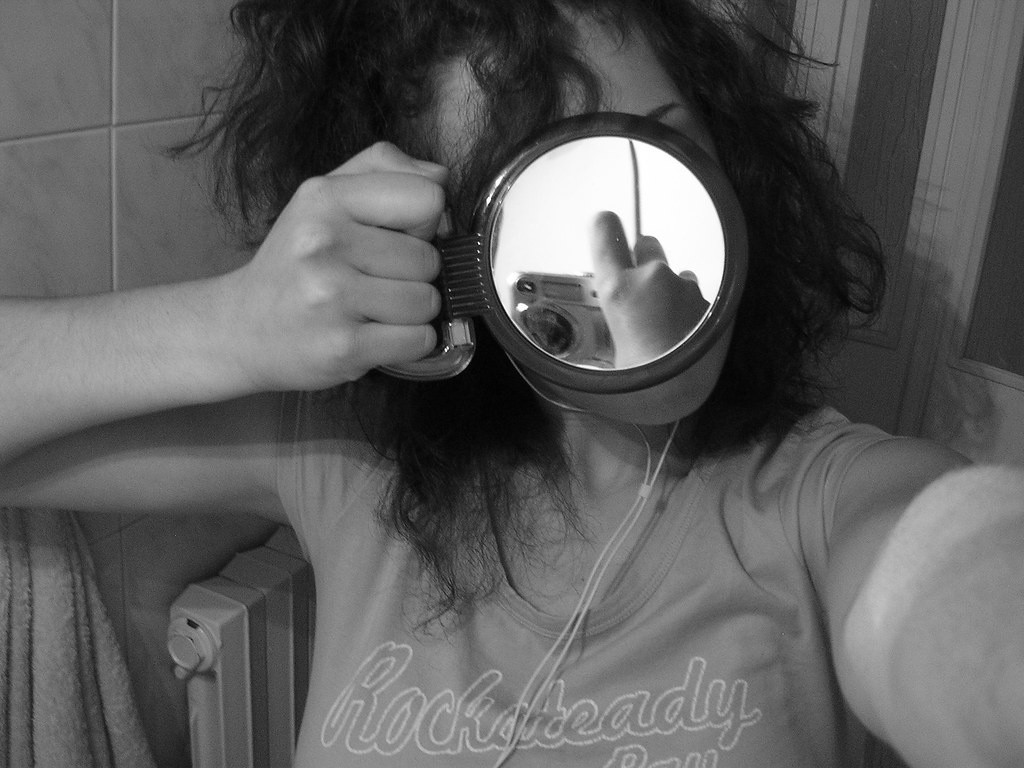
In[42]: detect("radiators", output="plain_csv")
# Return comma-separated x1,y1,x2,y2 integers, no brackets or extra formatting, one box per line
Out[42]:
167,525,316,768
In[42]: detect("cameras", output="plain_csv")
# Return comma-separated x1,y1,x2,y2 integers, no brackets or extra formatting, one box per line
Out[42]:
511,274,614,368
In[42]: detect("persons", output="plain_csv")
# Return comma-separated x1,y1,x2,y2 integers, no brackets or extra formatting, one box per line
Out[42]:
0,0,1024,767
589,211,711,369
